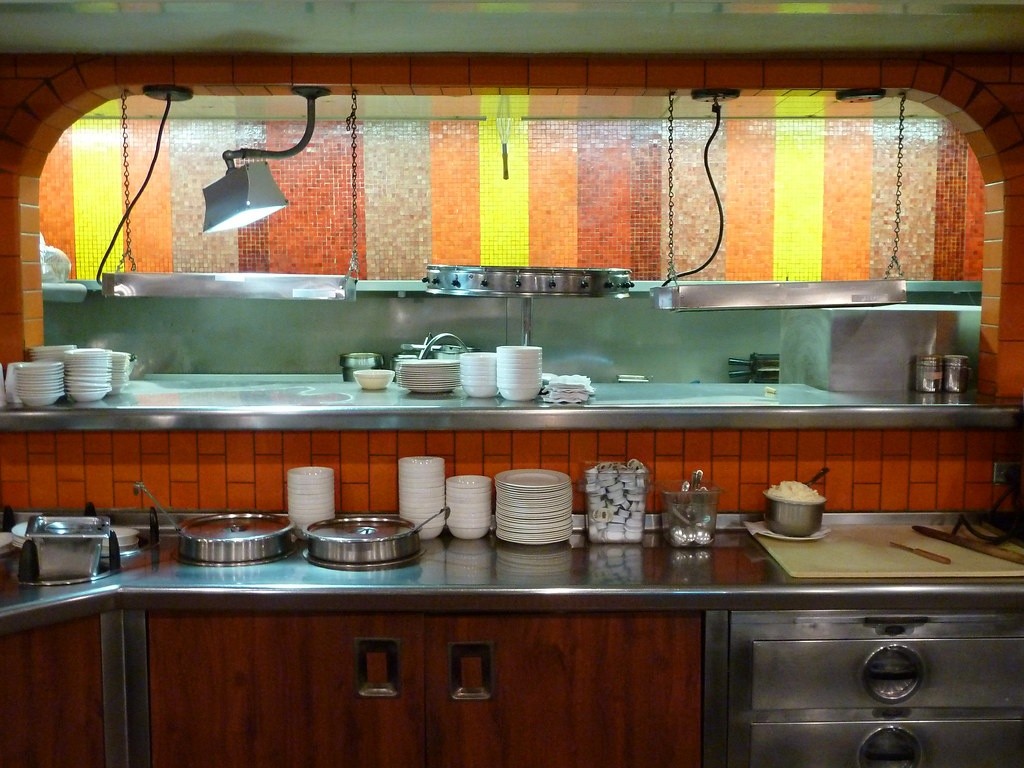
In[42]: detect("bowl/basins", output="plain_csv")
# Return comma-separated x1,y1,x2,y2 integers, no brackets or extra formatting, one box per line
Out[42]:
286,466,336,540
15,344,131,407
580,463,651,544
397,456,493,539
101,527,140,557
460,346,543,401
353,369,395,389
659,479,723,547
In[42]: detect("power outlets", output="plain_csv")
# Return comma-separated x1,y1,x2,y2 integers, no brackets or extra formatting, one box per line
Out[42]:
993,462,1022,484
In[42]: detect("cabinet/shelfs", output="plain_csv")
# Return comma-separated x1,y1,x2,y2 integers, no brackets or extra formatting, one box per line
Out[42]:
124,605,703,768
0,609,122,768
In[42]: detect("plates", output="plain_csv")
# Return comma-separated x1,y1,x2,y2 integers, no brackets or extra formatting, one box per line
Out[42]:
763,489,828,507
494,469,573,545
11,521,28,548
398,359,461,393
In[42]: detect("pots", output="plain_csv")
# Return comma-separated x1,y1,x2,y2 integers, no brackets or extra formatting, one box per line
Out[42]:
762,464,830,537
302,513,423,564
178,513,297,563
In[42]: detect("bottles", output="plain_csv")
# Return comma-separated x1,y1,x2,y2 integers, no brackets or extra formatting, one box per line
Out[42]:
943,355,970,393
915,354,942,392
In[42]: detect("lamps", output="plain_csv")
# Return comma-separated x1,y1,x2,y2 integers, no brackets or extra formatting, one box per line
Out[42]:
646,88,910,312
200,84,335,233
99,84,359,303
